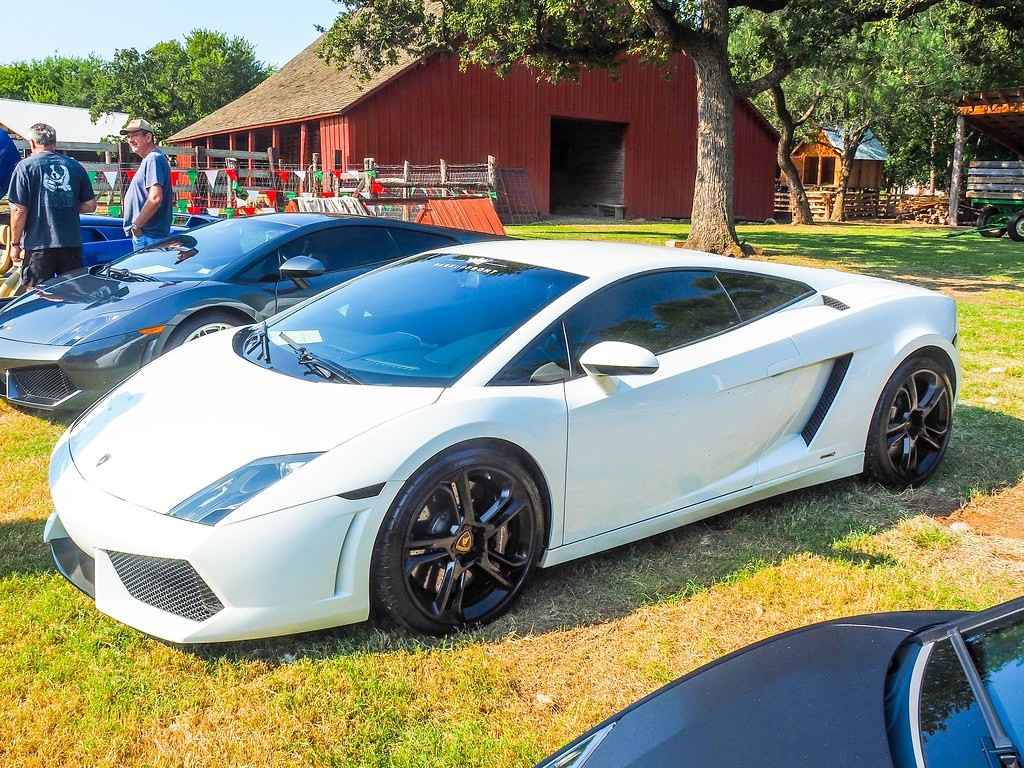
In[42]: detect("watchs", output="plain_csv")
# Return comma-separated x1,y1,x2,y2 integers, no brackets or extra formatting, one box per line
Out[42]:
131,223,138,230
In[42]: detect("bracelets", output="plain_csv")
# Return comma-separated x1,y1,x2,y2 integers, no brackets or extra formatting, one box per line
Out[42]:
10,242,22,247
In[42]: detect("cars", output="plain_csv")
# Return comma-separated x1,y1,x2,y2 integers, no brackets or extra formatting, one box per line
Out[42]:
42,235,959,645
0,212,526,412
531,594,1024,768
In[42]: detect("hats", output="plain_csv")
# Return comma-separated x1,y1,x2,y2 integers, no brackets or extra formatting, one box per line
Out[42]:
120,119,153,135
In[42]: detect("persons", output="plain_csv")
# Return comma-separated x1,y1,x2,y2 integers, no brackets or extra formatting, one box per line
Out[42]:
38,265,130,312
120,119,172,251
7,123,96,288
139,235,199,265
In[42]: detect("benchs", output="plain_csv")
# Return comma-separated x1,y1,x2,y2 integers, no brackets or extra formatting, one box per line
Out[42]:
595,203,626,220
